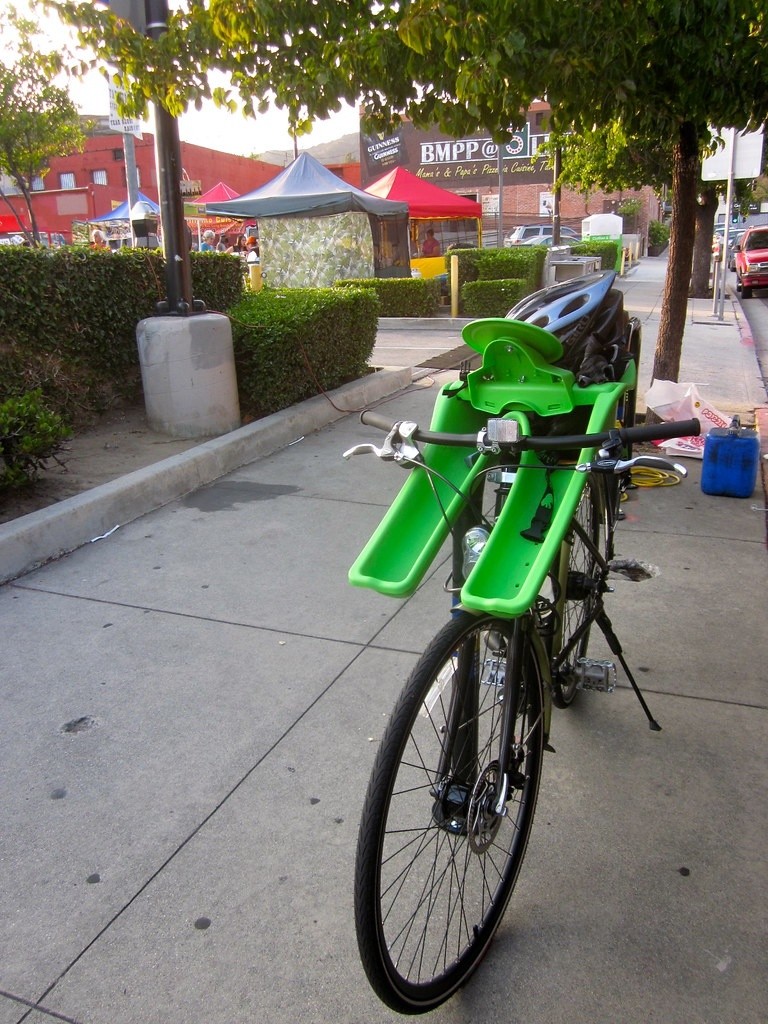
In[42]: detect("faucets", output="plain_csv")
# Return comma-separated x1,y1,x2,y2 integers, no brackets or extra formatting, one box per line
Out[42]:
559,250,568,255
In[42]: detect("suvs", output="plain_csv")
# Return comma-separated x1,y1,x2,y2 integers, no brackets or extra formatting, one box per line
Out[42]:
503,225,578,248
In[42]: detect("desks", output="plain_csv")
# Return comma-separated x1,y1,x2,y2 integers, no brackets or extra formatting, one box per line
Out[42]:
411,256,447,279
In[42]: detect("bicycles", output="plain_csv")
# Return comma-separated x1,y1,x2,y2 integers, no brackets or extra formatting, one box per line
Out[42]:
342,271,703,1015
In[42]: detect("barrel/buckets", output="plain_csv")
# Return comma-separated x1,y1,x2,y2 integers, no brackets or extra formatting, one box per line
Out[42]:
701,414,760,498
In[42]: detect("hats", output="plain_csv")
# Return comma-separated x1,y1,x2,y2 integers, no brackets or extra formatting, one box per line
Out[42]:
247,236,256,245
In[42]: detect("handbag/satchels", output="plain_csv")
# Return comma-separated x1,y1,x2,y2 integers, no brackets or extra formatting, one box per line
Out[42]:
644,378,732,447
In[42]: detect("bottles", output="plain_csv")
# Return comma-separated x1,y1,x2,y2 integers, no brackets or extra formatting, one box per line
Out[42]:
537,576,556,618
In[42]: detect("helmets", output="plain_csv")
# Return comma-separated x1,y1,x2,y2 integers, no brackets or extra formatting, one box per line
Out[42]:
504,268,629,386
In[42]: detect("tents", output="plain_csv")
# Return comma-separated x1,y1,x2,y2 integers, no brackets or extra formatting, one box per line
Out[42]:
90,151,484,288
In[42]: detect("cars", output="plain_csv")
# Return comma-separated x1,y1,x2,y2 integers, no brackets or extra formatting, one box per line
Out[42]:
714,223,768,299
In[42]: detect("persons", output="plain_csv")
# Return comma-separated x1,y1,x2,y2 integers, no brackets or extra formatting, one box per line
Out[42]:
24,230,260,278
413,228,441,258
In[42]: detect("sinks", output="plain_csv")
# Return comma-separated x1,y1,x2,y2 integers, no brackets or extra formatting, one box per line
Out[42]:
570,256,602,269
552,260,594,282
